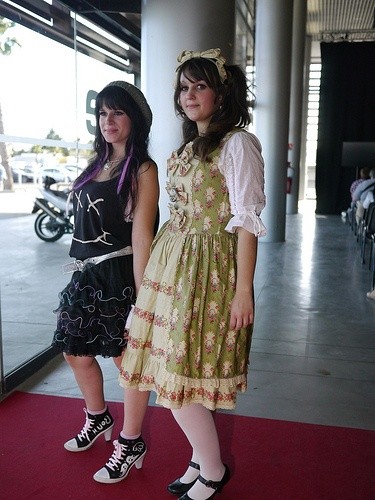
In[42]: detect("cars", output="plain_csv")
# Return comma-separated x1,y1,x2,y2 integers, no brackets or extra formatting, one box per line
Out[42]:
1,154,90,185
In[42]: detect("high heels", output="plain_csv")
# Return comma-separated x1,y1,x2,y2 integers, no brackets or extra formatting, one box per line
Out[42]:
168,461,230,500
64,405,115,452
92,433,148,483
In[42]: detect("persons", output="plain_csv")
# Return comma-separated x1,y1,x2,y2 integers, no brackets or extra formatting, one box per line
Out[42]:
49,80,160,485
349,167,375,228
117,47,267,500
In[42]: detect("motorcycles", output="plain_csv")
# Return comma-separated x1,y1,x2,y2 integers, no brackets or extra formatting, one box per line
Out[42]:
31,176,77,244
